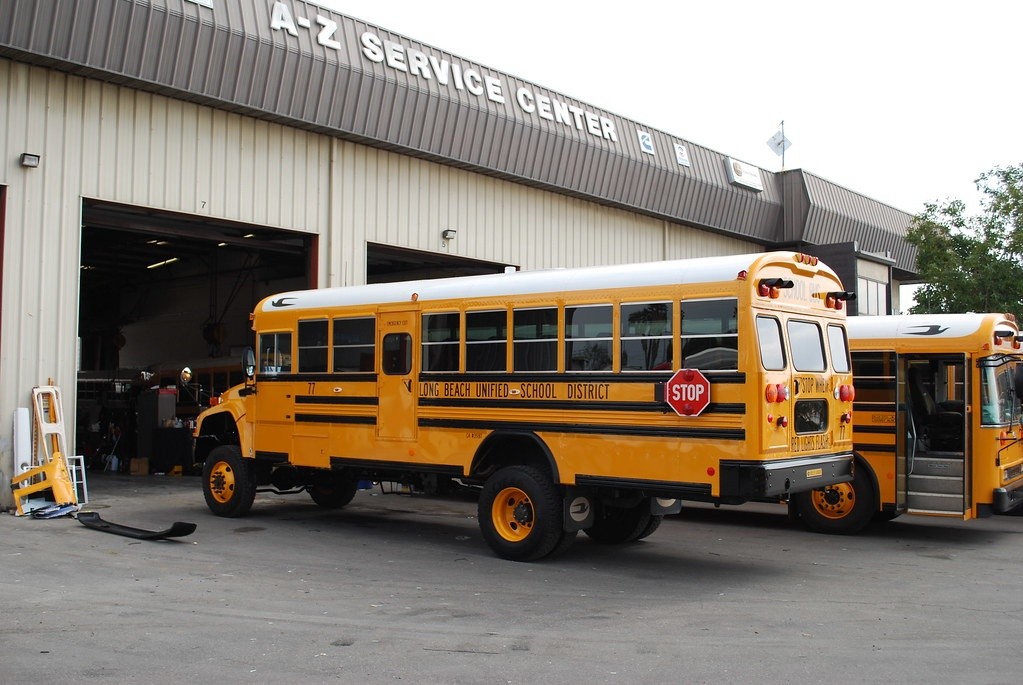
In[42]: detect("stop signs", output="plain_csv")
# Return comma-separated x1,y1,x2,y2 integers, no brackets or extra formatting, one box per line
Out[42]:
666,368,710,417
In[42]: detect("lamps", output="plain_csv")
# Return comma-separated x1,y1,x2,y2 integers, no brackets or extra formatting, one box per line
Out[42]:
443,230,456,240
20,153,40,168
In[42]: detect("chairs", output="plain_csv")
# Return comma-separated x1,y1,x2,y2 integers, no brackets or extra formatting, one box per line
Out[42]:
478,336,521,371
587,332,628,370
909,366,962,427
430,337,471,370
682,338,718,360
534,335,573,370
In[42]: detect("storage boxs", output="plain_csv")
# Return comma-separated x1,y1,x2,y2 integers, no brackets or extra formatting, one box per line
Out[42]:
130,457,148,475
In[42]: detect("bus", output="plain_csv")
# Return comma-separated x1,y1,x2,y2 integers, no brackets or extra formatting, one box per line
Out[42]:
190,250,854,564
133,353,295,419
774,313,1023,536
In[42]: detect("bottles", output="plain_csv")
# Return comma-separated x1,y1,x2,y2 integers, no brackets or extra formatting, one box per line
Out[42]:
111,455,118,471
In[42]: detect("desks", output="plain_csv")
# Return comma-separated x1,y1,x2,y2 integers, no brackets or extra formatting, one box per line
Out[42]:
152,428,193,472
